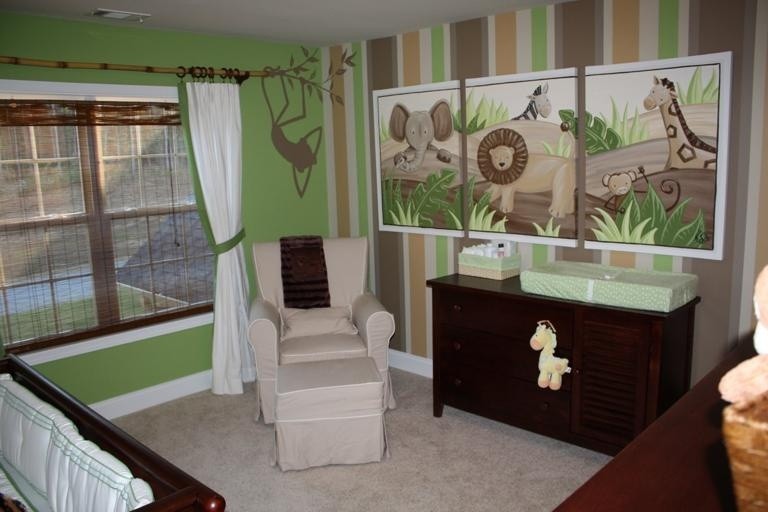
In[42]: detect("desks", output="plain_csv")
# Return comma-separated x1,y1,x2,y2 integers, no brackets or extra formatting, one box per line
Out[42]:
548,331,768,510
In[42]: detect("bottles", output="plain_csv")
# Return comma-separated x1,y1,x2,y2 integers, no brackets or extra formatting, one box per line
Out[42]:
463,241,516,259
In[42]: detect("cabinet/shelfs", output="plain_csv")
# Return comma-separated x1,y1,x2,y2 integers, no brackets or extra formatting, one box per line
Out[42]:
426,273,702,459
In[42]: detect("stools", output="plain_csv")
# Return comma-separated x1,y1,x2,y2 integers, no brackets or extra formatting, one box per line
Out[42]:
269,356,384,471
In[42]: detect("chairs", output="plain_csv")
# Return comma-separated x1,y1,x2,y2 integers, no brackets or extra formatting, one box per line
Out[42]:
248,236,396,471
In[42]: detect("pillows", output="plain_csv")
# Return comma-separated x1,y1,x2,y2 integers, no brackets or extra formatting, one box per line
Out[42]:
279,306,359,343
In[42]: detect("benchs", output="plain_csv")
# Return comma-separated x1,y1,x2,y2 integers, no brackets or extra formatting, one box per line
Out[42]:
1,353,226,511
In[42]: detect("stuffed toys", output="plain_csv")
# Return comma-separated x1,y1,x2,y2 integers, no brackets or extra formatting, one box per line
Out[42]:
530,320,571,390
